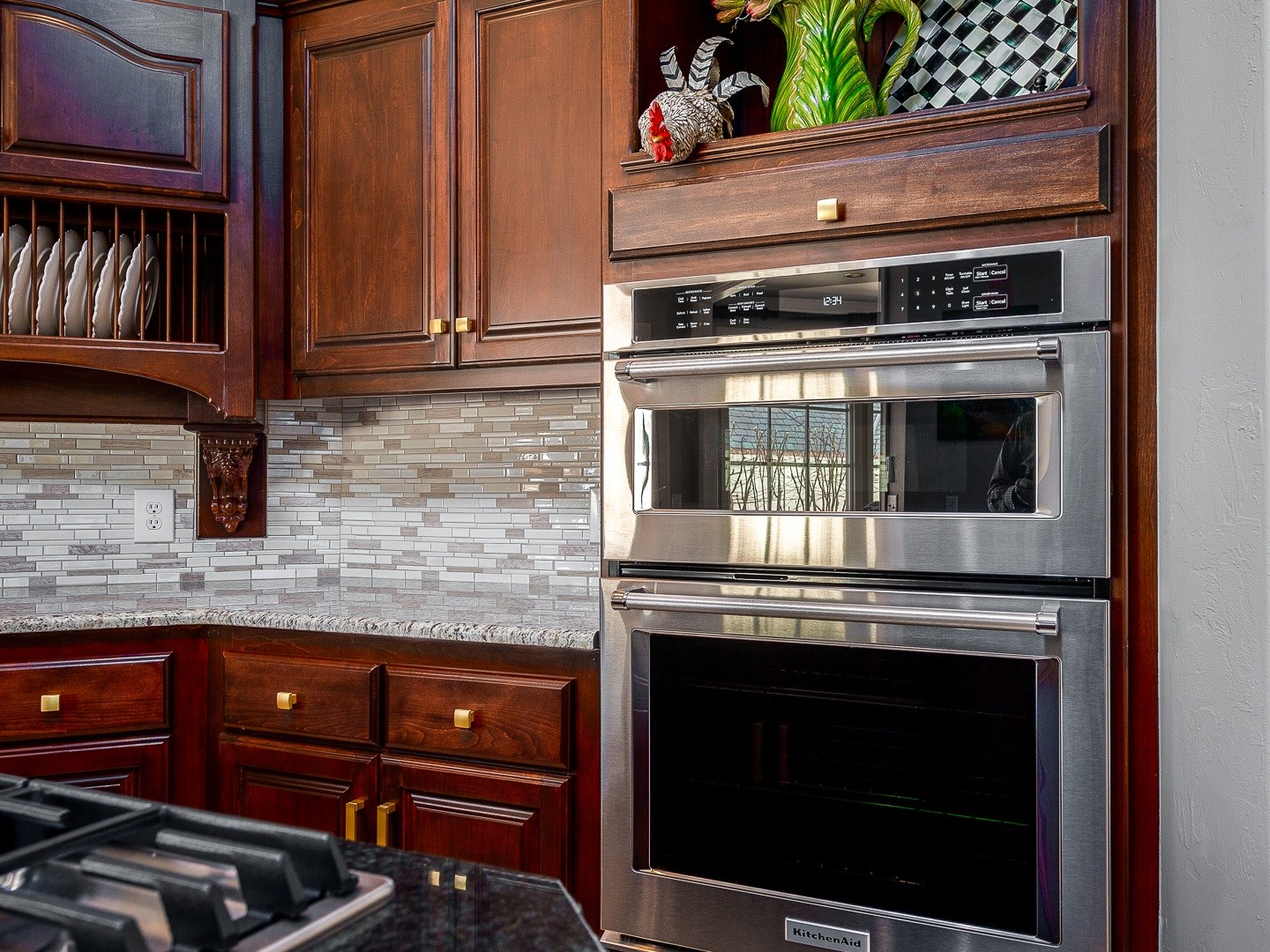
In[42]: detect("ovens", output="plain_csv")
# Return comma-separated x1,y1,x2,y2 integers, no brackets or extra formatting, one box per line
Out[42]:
601,236,1112,576
598,577,1112,951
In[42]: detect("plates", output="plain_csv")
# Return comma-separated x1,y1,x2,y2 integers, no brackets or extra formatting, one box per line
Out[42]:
8,225,55,335
91,233,133,338
36,228,82,335
0,224,28,325
117,235,160,339
63,230,108,338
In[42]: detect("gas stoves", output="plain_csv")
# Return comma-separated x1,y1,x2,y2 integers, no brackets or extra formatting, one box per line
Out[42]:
0,773,396,951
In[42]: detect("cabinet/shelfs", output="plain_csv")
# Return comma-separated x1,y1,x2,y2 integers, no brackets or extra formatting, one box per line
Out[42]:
0,0,1150,425
0,637,602,917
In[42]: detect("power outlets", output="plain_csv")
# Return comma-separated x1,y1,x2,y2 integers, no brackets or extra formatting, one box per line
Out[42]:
134,488,175,544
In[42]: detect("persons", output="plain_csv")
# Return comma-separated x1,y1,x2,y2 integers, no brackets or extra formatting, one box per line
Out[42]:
987,408,1036,513
